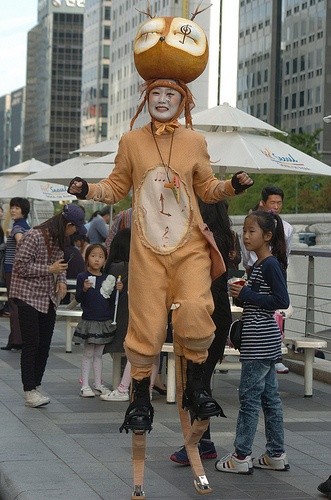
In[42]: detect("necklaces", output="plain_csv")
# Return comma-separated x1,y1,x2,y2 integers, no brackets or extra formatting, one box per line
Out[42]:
151,121,180,204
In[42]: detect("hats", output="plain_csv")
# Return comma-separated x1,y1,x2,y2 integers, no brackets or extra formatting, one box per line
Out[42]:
72,225,88,241
63,203,85,226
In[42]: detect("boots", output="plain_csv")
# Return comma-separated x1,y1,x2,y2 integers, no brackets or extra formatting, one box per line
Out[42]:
182,358,218,413
128,377,153,423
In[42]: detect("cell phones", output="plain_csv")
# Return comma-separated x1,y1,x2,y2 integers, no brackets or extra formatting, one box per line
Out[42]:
63,256,72,263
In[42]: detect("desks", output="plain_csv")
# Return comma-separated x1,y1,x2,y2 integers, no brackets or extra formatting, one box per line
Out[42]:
171,297,294,389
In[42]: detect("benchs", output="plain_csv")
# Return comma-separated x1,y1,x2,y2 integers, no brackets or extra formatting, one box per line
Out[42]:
112,338,328,404
55,310,83,353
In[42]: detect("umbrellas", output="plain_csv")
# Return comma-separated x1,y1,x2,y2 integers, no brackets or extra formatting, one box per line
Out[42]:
83,102,331,181
18,139,121,231
0,158,77,229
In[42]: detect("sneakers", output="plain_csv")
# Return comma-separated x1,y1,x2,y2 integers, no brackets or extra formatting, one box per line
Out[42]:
252,452,290,471
93,383,111,396
213,454,253,474
169,441,217,464
25,385,52,408
99,388,129,401
80,385,95,398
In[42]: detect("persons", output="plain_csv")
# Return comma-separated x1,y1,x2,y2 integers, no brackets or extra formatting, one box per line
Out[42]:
214,210,289,473
67,77,253,434
8,203,86,407
227,186,294,372
0,197,31,350
170,196,233,463
64,204,168,402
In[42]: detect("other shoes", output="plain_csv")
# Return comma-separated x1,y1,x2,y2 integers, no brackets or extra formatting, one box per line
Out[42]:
153,385,167,395
274,362,289,373
1,343,23,351
